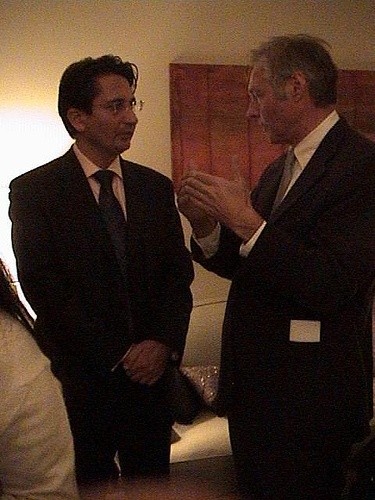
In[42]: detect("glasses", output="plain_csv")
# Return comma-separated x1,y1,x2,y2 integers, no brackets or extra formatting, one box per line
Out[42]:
78,100,145,117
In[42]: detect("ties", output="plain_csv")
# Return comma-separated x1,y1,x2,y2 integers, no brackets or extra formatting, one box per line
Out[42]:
270,150,295,217
93,169,128,281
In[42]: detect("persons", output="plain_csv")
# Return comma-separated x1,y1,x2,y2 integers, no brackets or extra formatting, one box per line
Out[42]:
177,32,374,500
7,52,195,482
0,257,79,500
